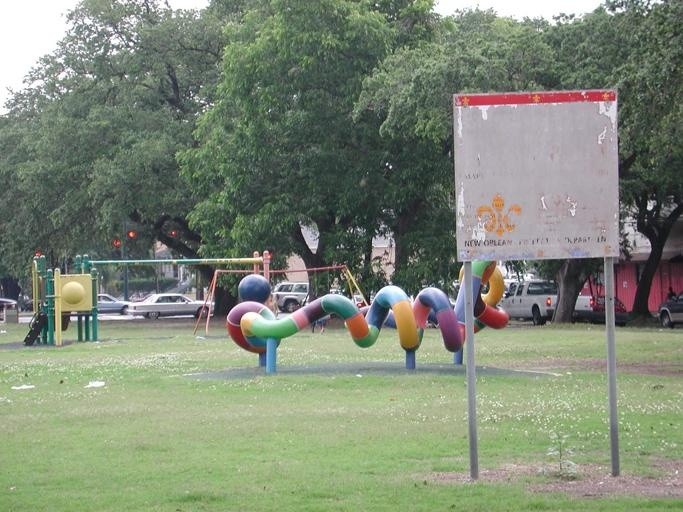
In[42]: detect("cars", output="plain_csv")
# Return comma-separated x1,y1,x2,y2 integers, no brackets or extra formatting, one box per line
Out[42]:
658,291,683,328
0,298,17,320
129,292,216,321
591,295,627,314
96,294,133,315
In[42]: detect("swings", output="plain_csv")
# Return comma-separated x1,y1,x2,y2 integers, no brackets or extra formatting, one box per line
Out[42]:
314,267,331,326
255,270,275,318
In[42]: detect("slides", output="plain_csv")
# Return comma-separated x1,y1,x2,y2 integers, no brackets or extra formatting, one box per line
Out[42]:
52,311,70,331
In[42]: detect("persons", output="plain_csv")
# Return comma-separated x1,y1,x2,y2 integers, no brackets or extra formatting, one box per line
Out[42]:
664,286,675,299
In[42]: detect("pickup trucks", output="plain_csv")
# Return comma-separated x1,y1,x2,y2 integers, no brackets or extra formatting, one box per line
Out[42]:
502,278,594,325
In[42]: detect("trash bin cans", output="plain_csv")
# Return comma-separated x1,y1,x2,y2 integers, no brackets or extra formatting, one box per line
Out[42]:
3,303,18,323
